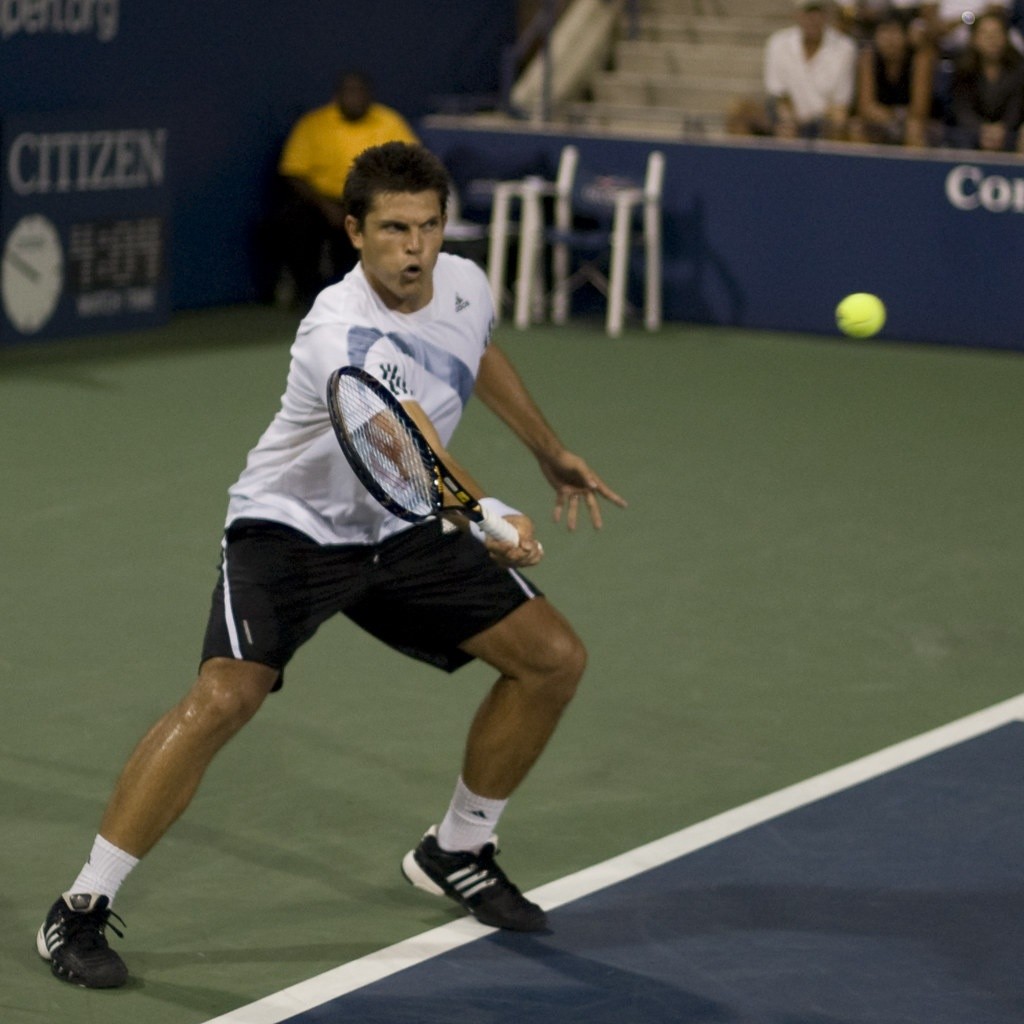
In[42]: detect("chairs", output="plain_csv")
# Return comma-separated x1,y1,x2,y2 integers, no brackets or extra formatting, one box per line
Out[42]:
444,144,674,336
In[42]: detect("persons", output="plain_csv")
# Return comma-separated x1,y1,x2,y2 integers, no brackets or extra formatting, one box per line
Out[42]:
280,73,419,225
36,137,629,987
721,0,1024,153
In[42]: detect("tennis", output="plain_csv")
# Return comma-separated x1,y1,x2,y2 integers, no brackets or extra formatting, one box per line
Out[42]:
835,292,886,339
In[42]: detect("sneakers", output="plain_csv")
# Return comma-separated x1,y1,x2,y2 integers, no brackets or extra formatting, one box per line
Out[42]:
401,825,547,933
37,892,129,987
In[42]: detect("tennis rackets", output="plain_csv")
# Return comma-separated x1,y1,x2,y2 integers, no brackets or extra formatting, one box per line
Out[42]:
326,365,544,559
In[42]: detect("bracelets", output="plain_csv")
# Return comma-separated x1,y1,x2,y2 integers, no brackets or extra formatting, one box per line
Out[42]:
469,497,524,543
